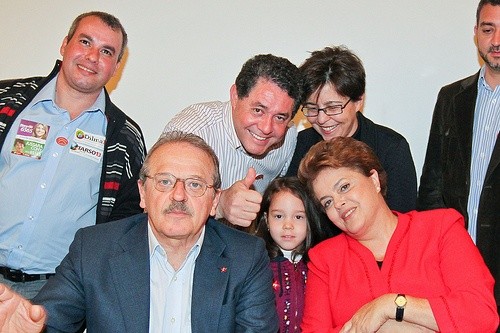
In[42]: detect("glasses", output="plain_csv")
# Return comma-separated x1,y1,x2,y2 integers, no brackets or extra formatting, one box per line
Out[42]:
301,97,351,116
144,172,214,196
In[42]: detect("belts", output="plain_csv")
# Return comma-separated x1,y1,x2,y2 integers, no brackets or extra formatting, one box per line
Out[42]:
0,265,56,282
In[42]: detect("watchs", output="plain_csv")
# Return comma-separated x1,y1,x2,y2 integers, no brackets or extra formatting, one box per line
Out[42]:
394,294,407,322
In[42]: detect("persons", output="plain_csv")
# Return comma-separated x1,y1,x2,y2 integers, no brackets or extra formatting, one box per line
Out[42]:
0,128,280,333
159,53,304,232
297,137,499,333
285,42,418,245
417,0,500,333
246,175,335,333
33,123,48,139
11,138,26,155
0,11,146,332
